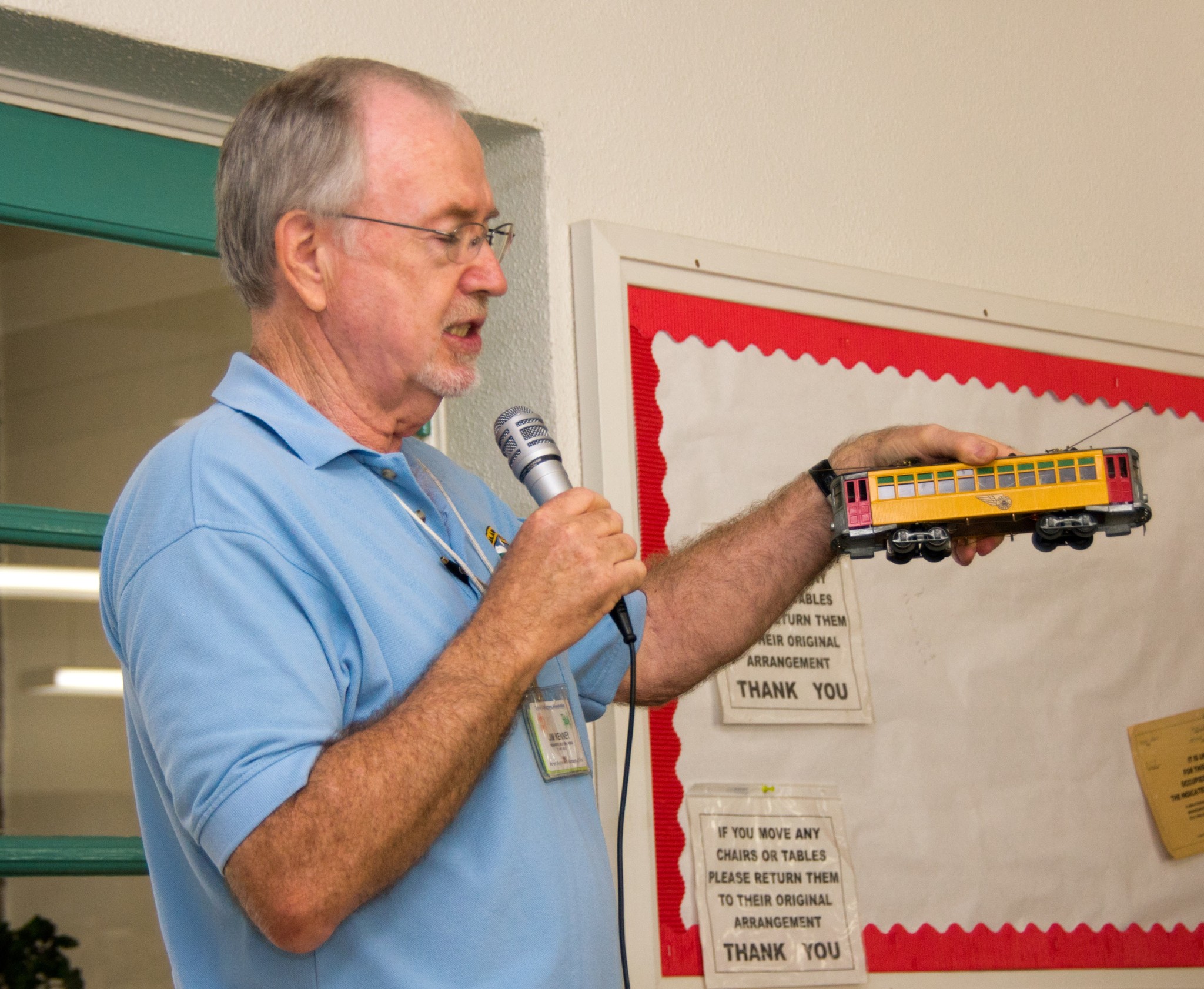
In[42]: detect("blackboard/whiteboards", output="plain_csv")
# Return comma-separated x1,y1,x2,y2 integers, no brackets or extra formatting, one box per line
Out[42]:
574,215,1204,989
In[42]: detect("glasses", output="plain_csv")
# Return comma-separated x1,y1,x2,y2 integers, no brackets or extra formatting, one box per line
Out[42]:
295,199,520,267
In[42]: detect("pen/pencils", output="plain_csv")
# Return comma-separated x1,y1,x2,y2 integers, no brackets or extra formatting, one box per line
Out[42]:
440,556,469,583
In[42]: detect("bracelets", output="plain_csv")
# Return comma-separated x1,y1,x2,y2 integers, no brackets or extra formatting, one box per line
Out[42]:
809,459,838,507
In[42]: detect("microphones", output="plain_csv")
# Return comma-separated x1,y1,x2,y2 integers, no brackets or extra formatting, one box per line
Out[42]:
494,406,638,644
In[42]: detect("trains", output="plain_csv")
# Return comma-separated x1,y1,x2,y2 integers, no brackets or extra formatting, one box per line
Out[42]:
828,446,1153,565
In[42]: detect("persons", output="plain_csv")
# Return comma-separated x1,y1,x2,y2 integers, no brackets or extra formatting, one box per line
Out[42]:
100,57,1030,989
